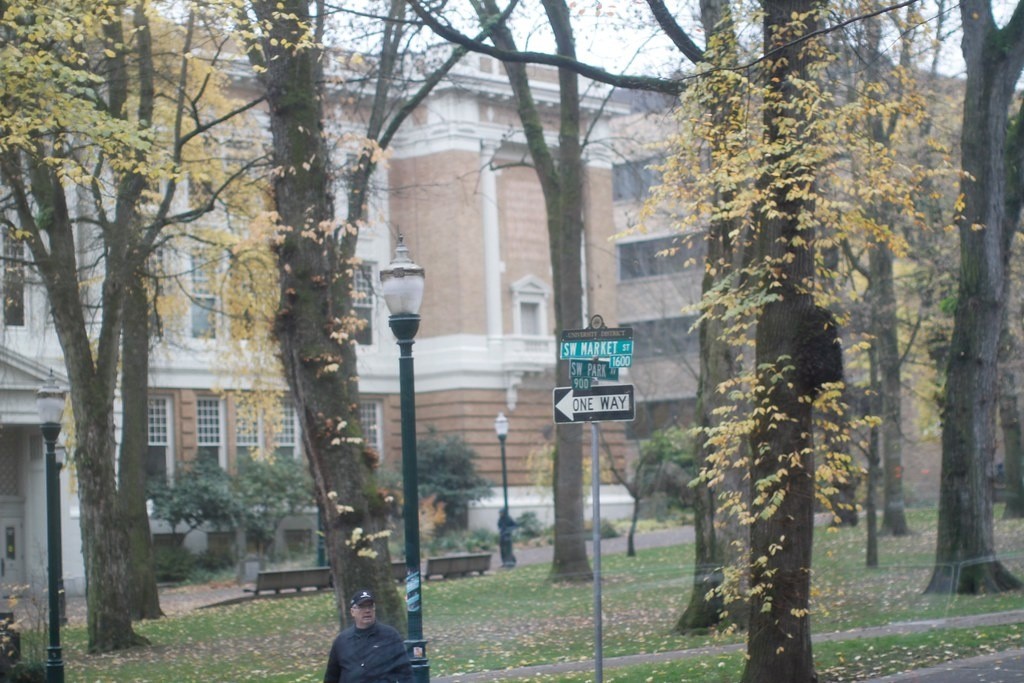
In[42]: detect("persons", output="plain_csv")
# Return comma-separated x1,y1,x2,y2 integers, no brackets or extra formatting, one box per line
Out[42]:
323,589,416,683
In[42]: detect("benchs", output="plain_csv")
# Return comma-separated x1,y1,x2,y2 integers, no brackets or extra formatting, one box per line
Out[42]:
243,566,331,597
392,562,409,583
421,554,493,580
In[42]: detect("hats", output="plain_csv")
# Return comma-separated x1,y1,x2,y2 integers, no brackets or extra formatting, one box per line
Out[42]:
350,590,376,608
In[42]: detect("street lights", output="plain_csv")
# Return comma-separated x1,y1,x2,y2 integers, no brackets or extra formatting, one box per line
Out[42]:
33,367,68,682
372,233,433,680
494,412,520,566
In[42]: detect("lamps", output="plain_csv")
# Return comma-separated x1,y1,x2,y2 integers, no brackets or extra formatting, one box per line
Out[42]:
56,440,66,472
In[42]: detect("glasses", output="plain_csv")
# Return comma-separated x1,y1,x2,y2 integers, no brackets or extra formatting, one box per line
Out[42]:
354,602,376,610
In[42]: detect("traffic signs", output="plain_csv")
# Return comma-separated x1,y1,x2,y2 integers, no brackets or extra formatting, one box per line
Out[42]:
552,385,636,426
